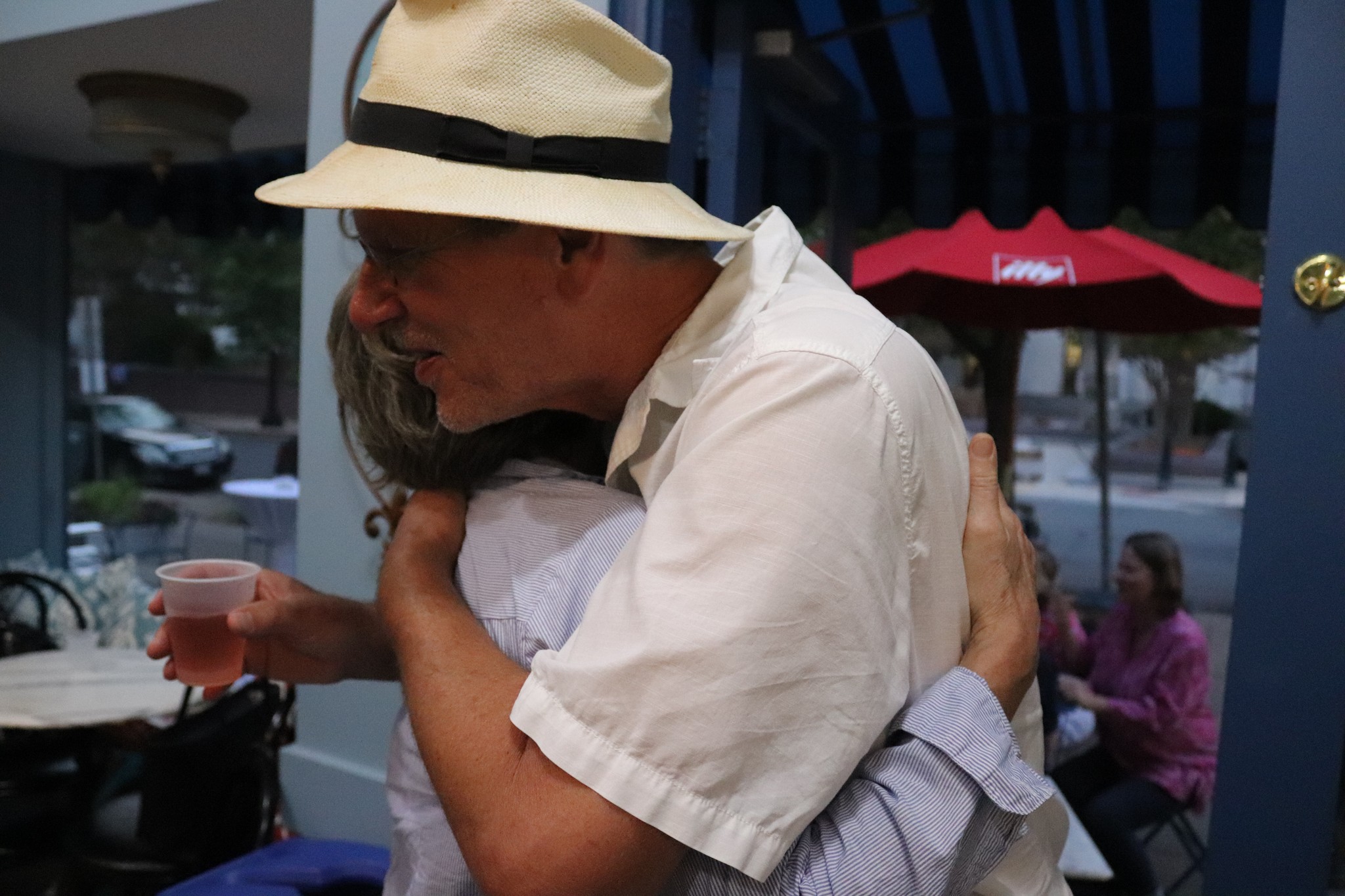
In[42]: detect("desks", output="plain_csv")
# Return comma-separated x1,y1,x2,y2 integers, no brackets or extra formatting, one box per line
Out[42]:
0,649,210,729
222,479,299,565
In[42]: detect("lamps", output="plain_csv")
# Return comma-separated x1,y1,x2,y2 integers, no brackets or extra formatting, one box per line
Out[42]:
77,71,251,180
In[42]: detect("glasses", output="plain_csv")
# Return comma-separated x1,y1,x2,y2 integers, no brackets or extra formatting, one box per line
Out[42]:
353,228,500,280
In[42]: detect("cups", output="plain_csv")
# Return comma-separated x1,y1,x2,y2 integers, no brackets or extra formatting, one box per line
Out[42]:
155,558,262,687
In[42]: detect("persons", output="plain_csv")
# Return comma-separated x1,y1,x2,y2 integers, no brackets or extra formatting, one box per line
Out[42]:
1058,530,1217,896
143,0,1086,896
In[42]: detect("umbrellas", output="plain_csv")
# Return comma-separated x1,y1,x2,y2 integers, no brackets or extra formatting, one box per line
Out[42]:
813,204,1262,514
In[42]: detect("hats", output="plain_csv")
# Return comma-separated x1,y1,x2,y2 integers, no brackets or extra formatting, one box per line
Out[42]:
253,0,757,242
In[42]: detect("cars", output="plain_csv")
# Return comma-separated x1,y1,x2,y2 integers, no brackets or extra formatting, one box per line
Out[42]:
67,394,231,491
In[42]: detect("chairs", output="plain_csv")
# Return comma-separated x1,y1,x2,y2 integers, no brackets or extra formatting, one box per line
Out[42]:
0,569,295,896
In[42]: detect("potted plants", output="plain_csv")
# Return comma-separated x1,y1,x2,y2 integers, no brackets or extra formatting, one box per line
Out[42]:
75,482,192,558
